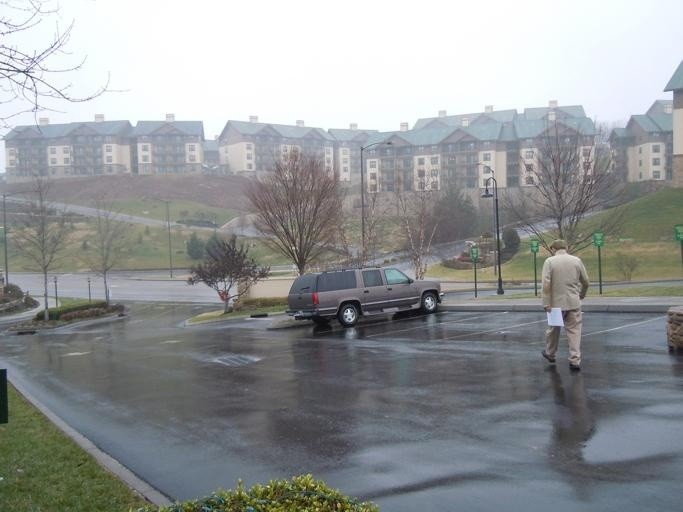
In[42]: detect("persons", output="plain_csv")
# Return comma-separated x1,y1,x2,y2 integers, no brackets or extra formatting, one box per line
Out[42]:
540,239,590,371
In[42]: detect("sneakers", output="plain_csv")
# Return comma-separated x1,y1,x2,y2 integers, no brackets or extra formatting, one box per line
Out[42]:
542,349,556,363
569,363,583,371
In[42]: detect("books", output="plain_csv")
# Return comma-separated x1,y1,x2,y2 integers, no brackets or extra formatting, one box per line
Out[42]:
546,308,564,327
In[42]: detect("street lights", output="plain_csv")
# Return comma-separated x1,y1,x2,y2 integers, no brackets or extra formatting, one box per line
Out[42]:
141,197,174,278
2,190,45,287
360,141,393,248
477,162,504,294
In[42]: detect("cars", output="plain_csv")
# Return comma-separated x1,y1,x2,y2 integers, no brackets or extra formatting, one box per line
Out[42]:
286,267,445,327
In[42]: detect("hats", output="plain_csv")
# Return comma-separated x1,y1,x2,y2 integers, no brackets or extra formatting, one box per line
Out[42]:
550,240,567,250
